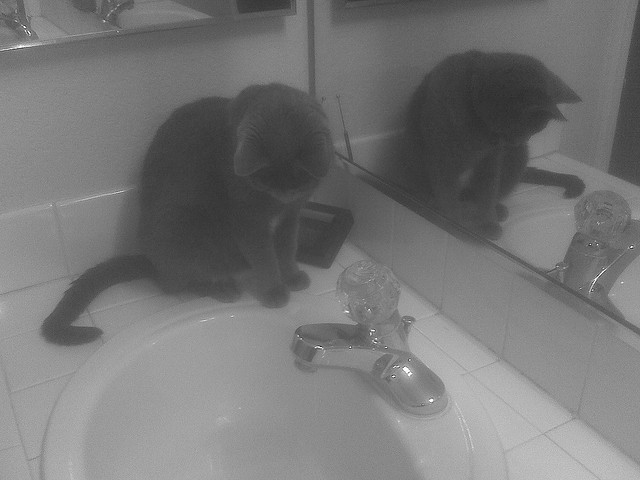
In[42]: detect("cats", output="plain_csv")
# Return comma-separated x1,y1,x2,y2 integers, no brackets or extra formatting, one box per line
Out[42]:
40,80,336,347
394,46,591,245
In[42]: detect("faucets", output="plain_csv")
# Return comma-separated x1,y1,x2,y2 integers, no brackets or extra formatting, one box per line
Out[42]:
544,191,640,298
291,260,448,417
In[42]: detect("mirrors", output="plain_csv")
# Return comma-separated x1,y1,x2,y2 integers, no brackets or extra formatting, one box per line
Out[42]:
307,0,640,337
0,0,299,57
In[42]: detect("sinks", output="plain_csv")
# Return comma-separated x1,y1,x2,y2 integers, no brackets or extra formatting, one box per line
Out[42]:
41,293,508,479
497,209,640,329
